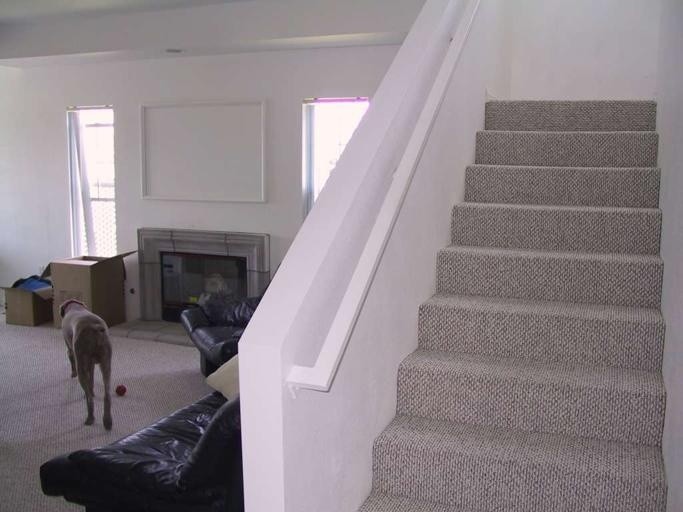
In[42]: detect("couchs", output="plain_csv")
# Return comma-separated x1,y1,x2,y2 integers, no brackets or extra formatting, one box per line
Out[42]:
41,391,244,511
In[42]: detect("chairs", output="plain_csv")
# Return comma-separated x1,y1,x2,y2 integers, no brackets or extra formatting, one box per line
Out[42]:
179,297,262,377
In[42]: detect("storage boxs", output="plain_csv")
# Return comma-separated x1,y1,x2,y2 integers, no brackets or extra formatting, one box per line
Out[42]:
41,251,137,328
1,280,52,327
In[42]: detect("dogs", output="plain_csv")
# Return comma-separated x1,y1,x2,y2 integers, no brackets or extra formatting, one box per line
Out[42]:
59,299,113,431
